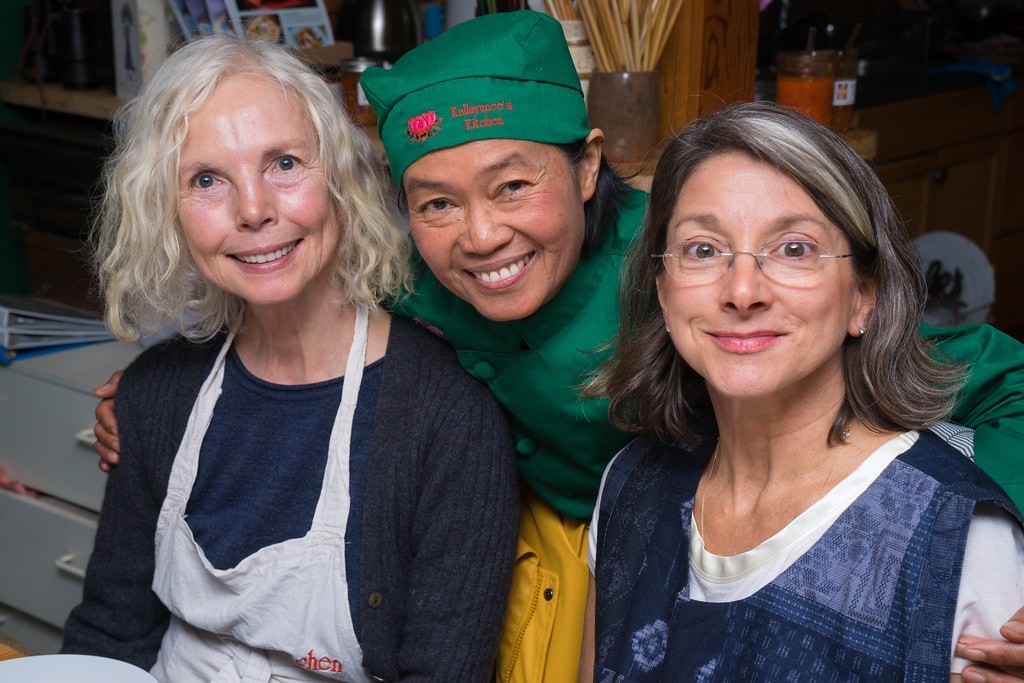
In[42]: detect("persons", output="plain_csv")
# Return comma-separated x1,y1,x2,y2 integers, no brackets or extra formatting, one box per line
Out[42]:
91,11,1024,683
581,102,1023,683
58,32,526,683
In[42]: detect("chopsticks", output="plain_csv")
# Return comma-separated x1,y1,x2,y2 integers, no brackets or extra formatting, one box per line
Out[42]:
542,0,683,73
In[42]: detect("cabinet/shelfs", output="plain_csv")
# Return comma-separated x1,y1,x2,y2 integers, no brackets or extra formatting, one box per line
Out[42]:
0,306,144,654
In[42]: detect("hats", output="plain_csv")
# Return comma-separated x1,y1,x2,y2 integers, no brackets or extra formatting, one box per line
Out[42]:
359,11,591,189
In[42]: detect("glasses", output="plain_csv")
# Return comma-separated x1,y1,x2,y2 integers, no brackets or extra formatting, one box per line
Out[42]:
651,239,858,284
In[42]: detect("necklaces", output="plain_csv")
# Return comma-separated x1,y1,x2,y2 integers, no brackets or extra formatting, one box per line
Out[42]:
700,416,855,545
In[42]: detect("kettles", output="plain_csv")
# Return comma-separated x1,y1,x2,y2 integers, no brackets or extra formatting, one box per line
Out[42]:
332,0,423,64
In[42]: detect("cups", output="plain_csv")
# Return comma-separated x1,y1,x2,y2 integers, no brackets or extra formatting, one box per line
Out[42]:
590,69,658,183
561,19,598,105
